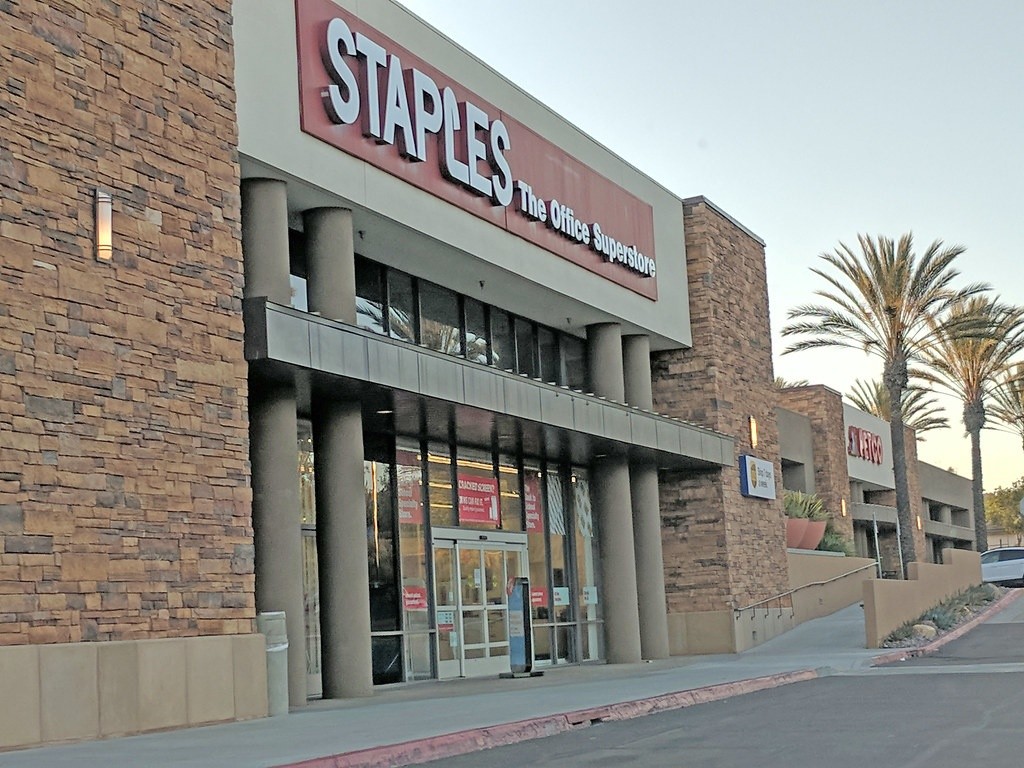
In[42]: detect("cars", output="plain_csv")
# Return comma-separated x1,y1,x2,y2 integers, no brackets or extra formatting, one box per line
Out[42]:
980,546,1024,588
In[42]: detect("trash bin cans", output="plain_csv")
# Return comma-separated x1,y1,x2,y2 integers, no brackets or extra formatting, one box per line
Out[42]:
256,610,289,716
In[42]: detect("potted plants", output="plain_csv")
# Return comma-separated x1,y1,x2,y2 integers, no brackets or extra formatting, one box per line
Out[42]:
783,488,811,546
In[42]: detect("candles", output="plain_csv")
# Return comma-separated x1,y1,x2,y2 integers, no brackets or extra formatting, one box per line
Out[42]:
798,493,838,549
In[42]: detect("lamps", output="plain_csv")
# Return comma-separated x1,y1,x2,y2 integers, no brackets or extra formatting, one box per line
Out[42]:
750,415,758,448
94,186,112,265
